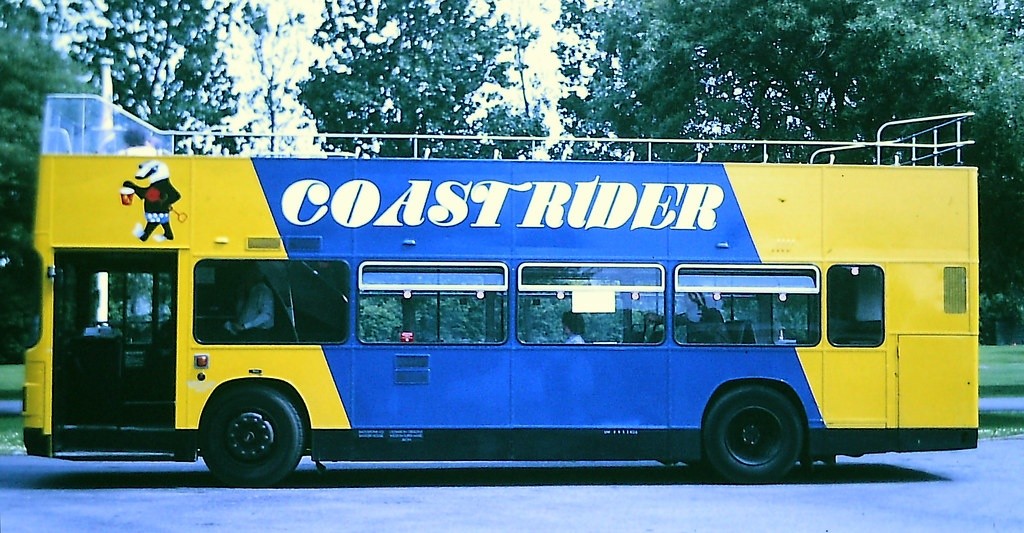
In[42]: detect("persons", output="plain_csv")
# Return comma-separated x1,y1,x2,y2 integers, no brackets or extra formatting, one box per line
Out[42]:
223,265,274,337
563,309,586,345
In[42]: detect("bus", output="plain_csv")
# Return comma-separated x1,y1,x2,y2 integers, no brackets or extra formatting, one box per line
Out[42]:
19,92,983,486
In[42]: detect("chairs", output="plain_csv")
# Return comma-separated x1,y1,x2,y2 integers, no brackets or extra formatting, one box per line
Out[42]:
630,321,756,346
